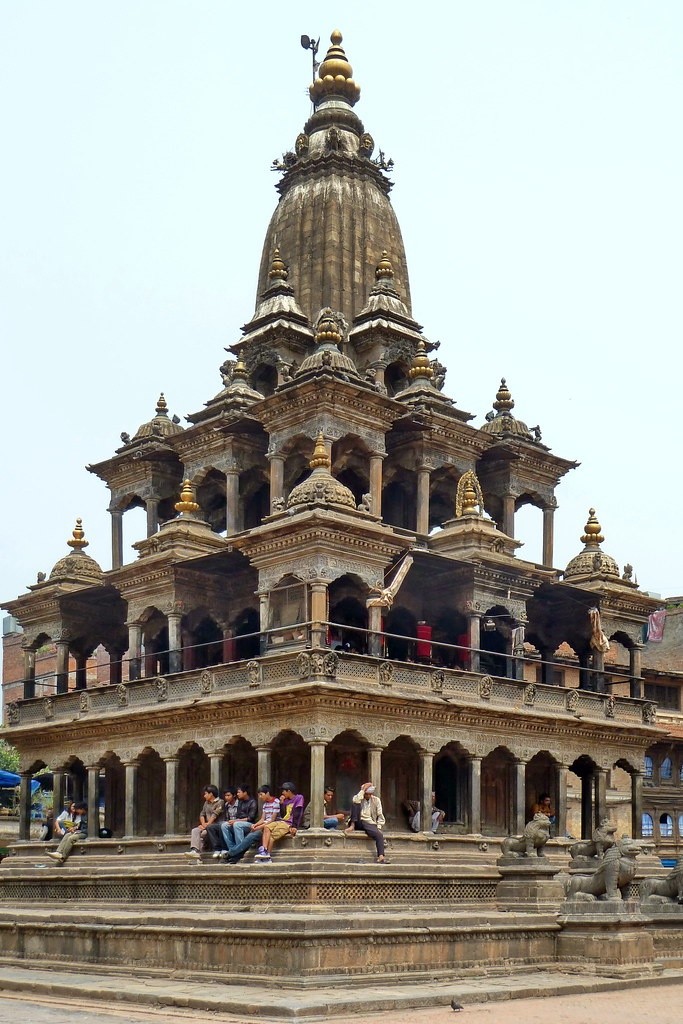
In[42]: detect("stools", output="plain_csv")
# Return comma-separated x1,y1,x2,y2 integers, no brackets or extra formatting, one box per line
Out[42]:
31,811,43,818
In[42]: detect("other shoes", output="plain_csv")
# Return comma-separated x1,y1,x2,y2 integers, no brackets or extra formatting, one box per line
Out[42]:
188,859,203,865
212,850,238,864
47,852,62,858
185,850,200,858
51,858,64,863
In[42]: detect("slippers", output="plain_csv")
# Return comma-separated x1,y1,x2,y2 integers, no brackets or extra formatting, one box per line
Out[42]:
376,858,391,864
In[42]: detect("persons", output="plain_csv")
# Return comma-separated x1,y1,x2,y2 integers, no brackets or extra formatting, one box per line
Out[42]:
254,782,304,862
38,807,54,841
206,787,239,858
185,785,223,858
334,642,488,675
402,790,445,833
45,802,88,862
223,785,281,864
54,802,76,838
531,794,556,837
303,786,344,830
346,783,392,864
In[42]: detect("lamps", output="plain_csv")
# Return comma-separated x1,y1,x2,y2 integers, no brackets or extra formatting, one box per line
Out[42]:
484,618,496,631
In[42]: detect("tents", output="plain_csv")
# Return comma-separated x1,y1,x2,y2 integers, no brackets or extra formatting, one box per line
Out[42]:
0,770,43,811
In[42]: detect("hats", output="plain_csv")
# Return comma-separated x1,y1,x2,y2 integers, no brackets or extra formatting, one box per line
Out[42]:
361,783,375,794
432,792,435,796
280,782,295,790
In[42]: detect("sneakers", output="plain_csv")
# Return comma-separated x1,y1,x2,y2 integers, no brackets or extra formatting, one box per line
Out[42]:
255,857,272,863
254,846,270,859
